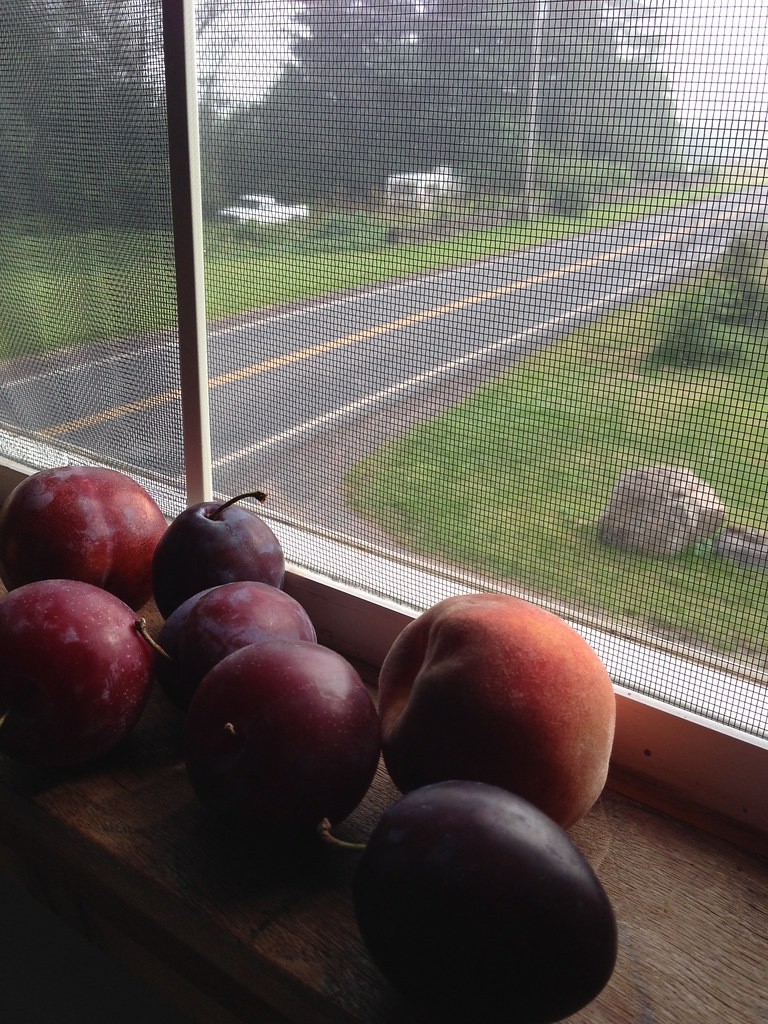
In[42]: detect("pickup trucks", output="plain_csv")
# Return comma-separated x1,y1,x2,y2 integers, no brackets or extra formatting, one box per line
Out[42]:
218,194,311,228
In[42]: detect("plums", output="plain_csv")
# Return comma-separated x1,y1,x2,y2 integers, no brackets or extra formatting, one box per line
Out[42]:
0,464,618,1024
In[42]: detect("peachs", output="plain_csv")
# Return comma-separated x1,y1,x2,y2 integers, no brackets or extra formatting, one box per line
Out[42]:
376,594,616,832
